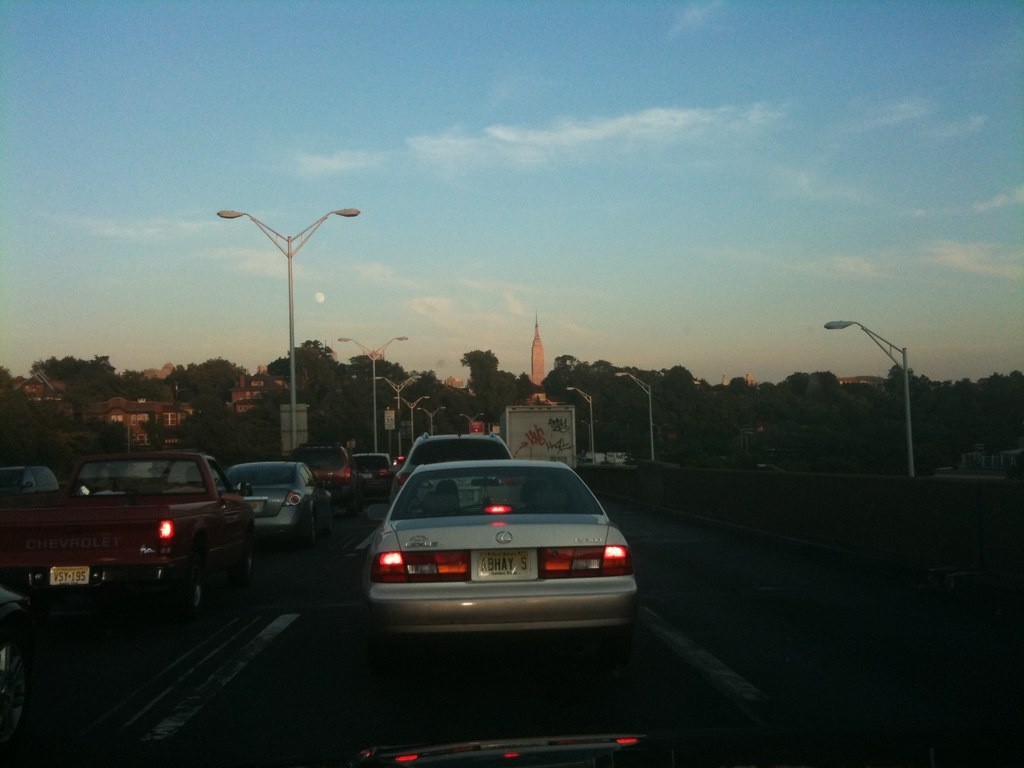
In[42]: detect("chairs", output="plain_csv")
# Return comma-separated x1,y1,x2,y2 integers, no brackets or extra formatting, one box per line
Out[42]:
421,495,459,514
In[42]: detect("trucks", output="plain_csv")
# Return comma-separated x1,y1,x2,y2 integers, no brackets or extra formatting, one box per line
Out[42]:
504,405,577,477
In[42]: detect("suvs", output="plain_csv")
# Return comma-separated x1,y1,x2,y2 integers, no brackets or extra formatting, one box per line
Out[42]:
387,431,519,518
292,443,360,515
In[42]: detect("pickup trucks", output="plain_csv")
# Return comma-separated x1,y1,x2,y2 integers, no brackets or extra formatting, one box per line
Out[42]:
0,448,256,621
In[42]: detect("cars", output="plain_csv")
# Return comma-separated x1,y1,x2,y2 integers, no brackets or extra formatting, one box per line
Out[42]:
211,460,332,547
0,584,39,767
362,458,640,657
0,465,58,501
348,450,401,498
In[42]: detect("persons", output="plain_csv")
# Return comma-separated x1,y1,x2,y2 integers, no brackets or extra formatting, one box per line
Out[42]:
434,479,461,510
186,466,244,502
423,491,449,514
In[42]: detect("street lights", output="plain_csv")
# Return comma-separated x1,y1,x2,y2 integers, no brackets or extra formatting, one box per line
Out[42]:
825,321,915,478
375,375,421,455
417,406,446,435
217,208,361,446
614,372,655,462
394,395,430,447
567,386,595,462
337,336,409,453
